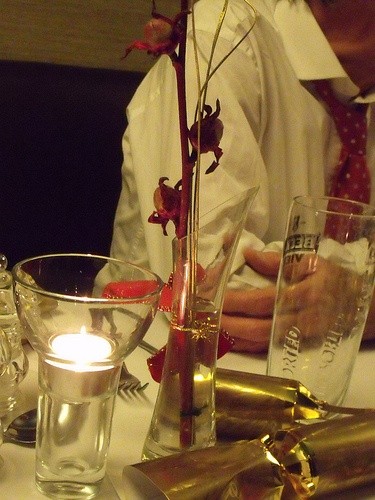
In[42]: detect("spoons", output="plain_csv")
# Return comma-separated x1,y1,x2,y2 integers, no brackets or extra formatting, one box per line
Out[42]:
5,407,36,444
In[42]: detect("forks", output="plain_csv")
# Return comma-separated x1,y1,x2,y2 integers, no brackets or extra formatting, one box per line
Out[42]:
118,360,149,391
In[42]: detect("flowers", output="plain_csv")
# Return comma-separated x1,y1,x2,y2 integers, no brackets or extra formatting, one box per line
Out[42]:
102,0,257,452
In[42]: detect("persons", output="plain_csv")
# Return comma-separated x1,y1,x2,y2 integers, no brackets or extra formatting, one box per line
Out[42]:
93,0,375,353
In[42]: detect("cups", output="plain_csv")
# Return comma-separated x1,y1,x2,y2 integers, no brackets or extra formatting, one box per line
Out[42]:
266,195,375,419
11,254,163,500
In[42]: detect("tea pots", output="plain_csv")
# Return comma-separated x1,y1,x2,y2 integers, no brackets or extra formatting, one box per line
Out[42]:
0,254,30,418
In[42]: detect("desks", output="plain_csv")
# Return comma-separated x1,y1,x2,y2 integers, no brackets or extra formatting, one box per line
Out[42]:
0,295,375,500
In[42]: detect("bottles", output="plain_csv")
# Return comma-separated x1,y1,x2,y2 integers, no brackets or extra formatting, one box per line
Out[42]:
141,186,262,462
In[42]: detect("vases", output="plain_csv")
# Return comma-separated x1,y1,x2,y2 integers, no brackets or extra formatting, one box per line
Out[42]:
140,187,260,462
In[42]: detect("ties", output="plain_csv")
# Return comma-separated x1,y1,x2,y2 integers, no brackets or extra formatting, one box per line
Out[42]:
306,81,371,244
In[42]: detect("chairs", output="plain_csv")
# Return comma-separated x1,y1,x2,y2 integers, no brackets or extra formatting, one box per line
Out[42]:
0,61,145,292
